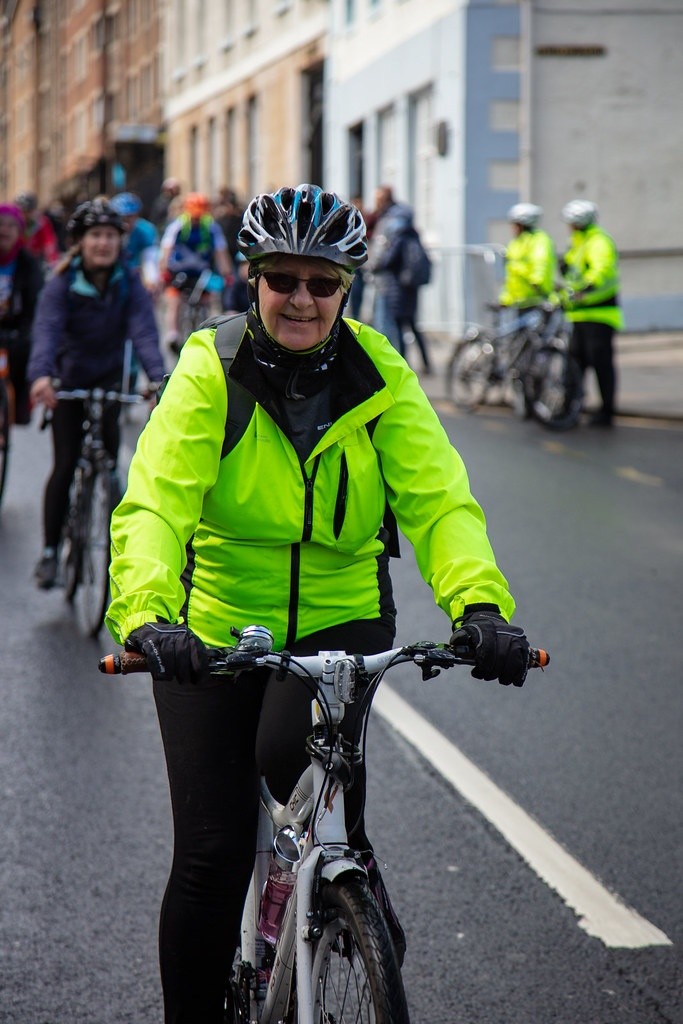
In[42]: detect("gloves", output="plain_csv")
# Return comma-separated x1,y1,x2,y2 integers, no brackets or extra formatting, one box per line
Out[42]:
125,615,210,681
449,603,530,688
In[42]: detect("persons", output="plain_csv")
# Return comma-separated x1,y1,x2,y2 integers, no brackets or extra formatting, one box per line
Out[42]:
113,177,250,350
345,187,430,372
548,198,624,427
495,203,557,413
105,184,532,1024
25,195,169,591
0,204,44,424
14,192,71,265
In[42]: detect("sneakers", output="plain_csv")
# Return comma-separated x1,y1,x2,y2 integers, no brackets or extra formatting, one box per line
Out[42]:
343,857,407,970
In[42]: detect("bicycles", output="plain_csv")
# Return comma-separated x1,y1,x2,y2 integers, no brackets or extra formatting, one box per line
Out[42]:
96,623,554,1024
444,280,589,432
168,280,234,332
40,388,152,644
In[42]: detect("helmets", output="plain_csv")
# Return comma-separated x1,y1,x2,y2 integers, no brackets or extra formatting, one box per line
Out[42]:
109,192,142,218
183,195,210,214
65,198,127,239
508,202,542,227
563,199,597,225
236,183,369,274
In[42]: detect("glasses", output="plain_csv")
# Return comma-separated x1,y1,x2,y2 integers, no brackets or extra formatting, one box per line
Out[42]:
255,270,344,298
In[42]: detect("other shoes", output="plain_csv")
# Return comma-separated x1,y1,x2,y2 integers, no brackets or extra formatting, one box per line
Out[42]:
35,557,58,581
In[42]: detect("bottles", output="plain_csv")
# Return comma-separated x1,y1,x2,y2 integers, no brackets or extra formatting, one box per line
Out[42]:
255,930,277,1002
258,822,303,945
